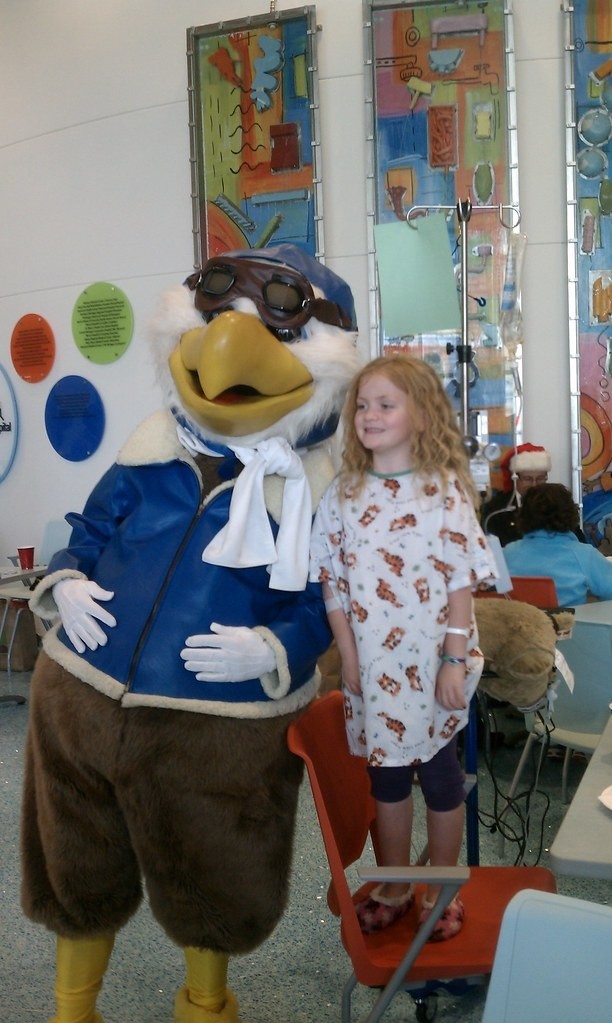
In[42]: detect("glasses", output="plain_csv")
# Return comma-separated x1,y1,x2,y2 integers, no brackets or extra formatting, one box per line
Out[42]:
517,472,549,484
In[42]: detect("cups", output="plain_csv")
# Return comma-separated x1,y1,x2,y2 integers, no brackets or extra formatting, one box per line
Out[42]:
17,546,35,570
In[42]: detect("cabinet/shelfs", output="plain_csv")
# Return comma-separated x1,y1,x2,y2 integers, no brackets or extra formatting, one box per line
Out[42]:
497,600,612,879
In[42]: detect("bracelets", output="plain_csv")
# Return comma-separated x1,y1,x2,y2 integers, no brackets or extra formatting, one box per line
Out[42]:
442,656,464,664
447,627,471,638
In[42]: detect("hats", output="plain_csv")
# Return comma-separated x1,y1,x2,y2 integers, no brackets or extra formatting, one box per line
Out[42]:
500,442,552,494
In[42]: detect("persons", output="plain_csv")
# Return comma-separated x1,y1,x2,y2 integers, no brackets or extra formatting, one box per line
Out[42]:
501,483,612,607
481,443,586,544
309,355,498,943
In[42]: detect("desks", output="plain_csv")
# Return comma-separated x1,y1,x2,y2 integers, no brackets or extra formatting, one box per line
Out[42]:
0,565,51,691
481,889,612,1022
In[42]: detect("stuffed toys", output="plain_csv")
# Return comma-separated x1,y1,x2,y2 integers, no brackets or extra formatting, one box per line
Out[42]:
21,243,363,1023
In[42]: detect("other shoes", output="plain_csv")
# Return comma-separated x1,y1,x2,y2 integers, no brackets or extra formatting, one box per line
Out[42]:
415,889,464,942
545,740,565,760
357,882,417,933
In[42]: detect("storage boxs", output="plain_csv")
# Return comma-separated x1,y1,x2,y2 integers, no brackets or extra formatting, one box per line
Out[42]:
0,600,38,672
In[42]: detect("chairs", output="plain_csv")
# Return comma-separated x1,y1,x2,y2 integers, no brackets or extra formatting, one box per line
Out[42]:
472,576,559,610
499,620,612,856
287,691,558,1023
